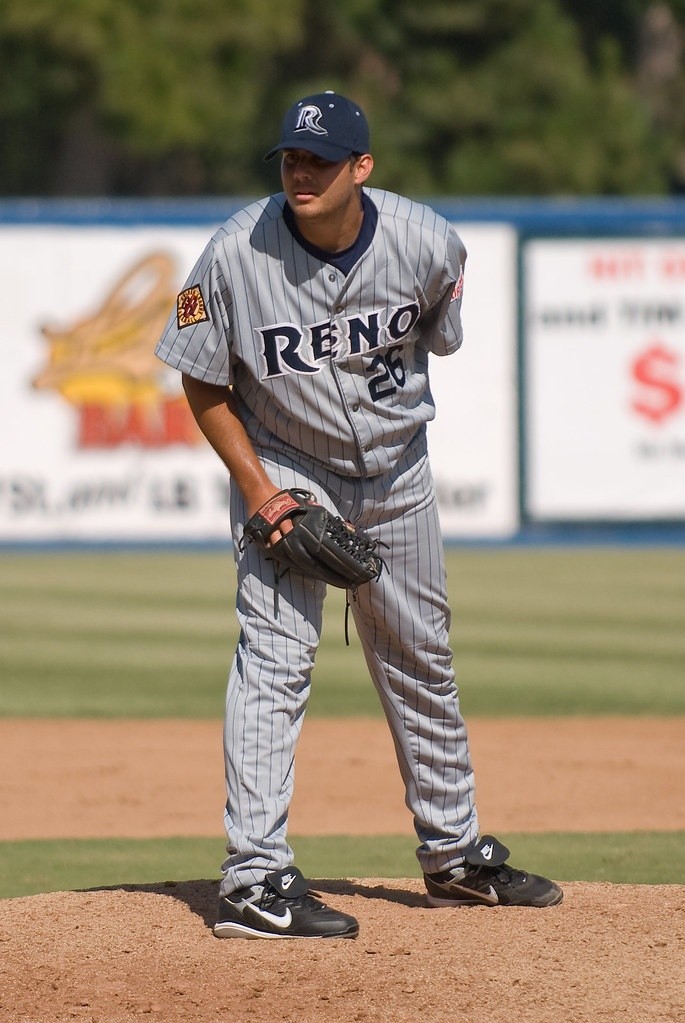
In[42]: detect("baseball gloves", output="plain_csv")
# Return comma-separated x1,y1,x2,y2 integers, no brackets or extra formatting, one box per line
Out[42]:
243,488,382,589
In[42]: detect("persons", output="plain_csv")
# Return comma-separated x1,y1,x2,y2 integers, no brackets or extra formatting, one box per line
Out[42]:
154,89,565,938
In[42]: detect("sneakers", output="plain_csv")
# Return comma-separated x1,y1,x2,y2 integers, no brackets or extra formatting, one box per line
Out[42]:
213,866,359,943
423,835,565,909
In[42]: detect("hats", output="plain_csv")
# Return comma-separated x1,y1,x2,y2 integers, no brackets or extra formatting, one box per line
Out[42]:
263,89,371,164
